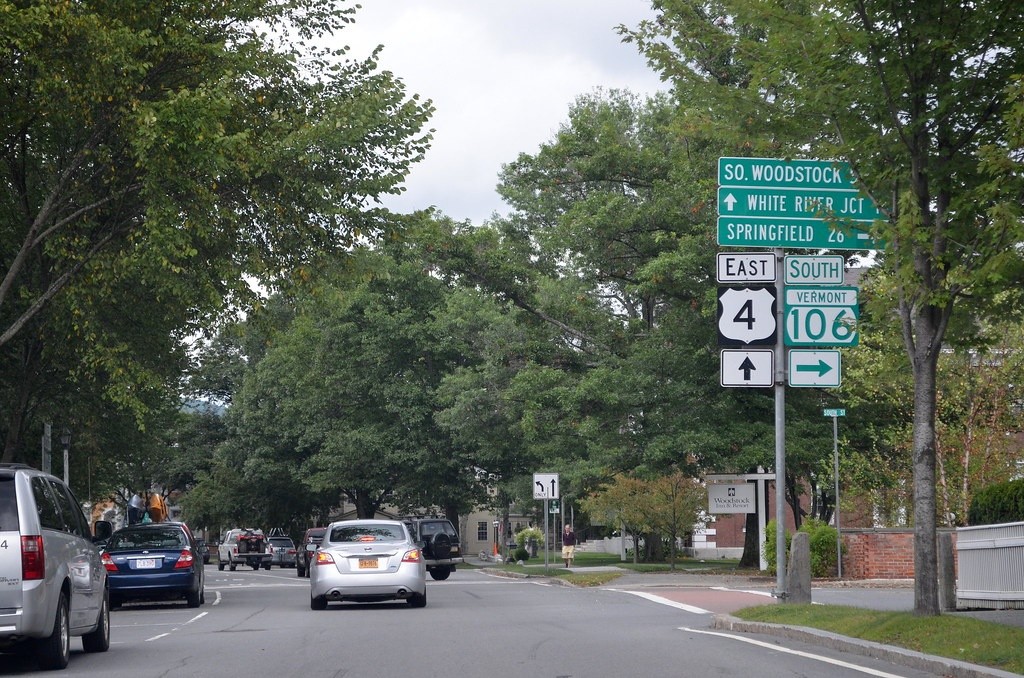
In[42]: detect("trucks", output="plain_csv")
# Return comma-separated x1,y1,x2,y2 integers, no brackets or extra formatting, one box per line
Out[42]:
218,527,274,571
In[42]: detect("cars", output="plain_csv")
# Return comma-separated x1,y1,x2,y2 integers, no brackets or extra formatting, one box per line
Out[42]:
193,537,211,564
99,521,208,609
294,526,327,578
264,536,297,568
308,519,428,611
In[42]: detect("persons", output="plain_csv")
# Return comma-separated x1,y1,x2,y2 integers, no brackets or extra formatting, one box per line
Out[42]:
561,524,575,568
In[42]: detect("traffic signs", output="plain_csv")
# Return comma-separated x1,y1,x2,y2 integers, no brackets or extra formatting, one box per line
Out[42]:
715,156,895,251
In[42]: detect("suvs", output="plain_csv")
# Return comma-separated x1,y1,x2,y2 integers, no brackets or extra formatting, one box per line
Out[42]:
399,516,464,581
0,462,115,671
